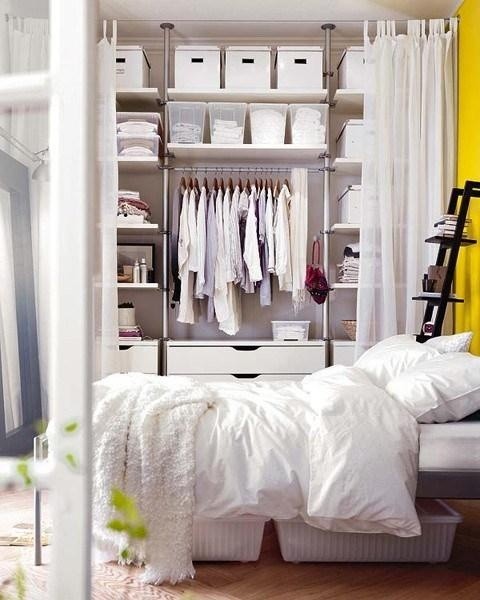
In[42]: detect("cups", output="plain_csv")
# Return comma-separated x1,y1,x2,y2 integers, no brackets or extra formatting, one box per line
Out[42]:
117,307,136,325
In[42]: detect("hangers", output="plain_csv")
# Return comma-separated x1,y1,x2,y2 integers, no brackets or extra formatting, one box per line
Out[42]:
172,164,291,198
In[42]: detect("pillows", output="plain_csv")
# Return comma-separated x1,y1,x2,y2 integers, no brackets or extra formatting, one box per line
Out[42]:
387,355,479,426
355,331,443,389
424,332,475,353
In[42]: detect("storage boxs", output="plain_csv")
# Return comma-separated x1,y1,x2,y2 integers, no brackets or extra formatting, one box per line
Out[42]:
270,320,312,343
116,44,151,89
245,99,287,146
173,44,223,89
165,100,207,143
272,497,466,564
187,514,271,565
117,135,163,160
337,184,361,224
336,44,364,91
221,46,274,89
202,101,246,144
275,45,324,92
333,119,362,156
286,102,331,145
116,111,165,139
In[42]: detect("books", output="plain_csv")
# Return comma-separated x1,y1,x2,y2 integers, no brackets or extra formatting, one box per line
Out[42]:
431,214,471,239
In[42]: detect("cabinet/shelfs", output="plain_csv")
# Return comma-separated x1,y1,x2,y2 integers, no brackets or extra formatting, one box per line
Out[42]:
160,19,327,383
105,17,169,377
323,17,463,367
409,180,480,344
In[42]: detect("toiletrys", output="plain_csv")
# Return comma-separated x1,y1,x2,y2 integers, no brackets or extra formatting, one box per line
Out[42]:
140,258,148,283
132,260,140,283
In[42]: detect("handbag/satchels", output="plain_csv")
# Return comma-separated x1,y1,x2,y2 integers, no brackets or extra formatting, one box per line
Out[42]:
304,239,333,305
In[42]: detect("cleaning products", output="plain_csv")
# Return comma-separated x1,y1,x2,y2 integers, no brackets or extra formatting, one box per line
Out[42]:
133,255,148,285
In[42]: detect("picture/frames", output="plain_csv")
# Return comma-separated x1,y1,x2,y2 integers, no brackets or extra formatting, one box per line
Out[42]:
115,242,154,282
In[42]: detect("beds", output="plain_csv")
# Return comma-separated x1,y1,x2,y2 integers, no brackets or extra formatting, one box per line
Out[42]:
30,331,479,568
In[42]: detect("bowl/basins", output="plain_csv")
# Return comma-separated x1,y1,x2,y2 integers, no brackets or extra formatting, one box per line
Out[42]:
341,318,356,340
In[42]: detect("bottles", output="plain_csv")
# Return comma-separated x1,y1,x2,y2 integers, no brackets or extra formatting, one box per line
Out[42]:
133,256,149,283
422,274,434,292
